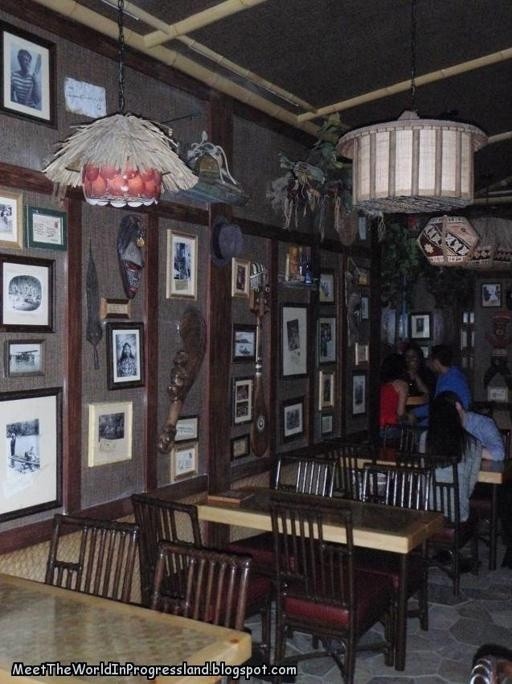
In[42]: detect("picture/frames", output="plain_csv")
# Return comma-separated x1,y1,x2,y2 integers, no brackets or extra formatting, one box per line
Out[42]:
480,281,503,309
486,384,509,402
1,17,60,131
420,343,431,358
407,310,434,341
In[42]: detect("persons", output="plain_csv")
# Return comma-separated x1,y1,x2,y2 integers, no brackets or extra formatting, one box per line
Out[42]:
24,445,39,471
176,243,191,280
377,352,418,441
434,390,506,463
410,343,472,426
115,341,138,379
10,432,17,466
401,339,436,400
417,399,484,574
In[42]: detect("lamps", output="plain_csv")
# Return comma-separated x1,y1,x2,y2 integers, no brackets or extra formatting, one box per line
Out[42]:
333,0,490,217
40,0,201,210
